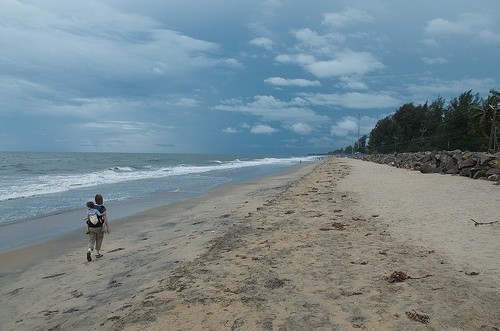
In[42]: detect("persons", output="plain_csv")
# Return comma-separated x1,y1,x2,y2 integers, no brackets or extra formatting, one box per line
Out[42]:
83,201,107,234
86,194,109,262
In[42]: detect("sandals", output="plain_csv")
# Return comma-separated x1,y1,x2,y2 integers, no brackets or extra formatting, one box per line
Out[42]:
95,254,103,258
87,251,91,261
